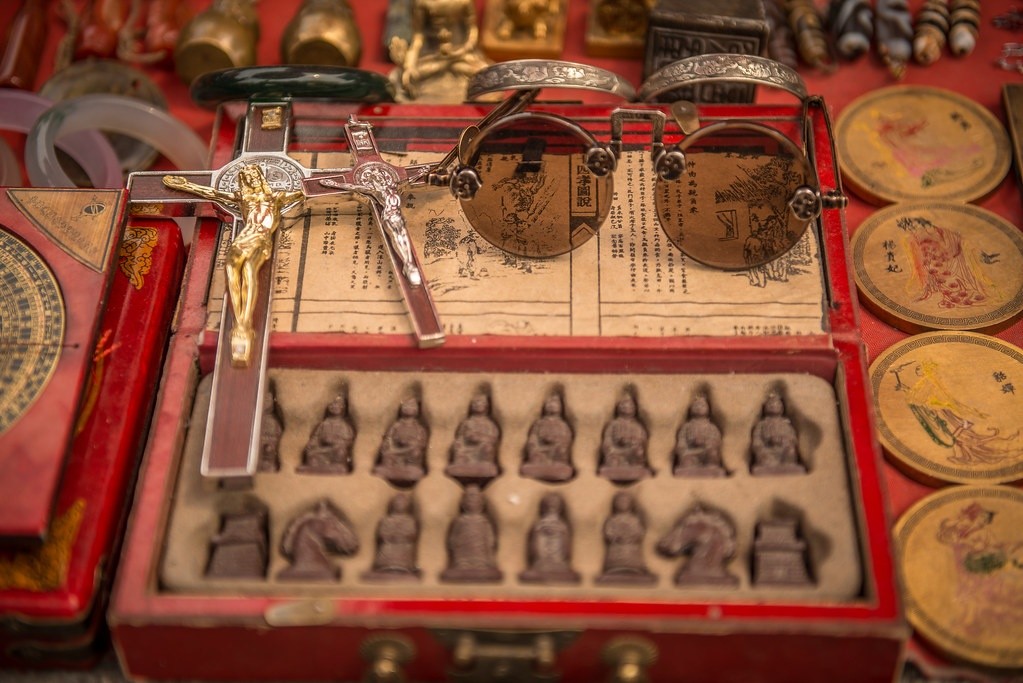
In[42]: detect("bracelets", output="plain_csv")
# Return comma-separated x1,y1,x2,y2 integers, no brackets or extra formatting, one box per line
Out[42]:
463,59,637,102
638,53,809,101
192,64,400,106
1,62,210,189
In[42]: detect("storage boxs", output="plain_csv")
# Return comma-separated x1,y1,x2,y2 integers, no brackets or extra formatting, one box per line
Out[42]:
104,97,909,683
0,216,183,675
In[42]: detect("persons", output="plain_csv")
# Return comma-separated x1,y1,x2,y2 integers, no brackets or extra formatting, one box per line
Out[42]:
527,392,572,464
304,394,355,467
159,163,304,365
257,391,284,466
450,393,498,467
320,164,432,287
402,0,479,99
447,485,495,569
752,393,797,464
380,398,427,470
528,495,572,571
603,495,649,574
602,393,647,466
675,395,723,468
375,493,417,575
